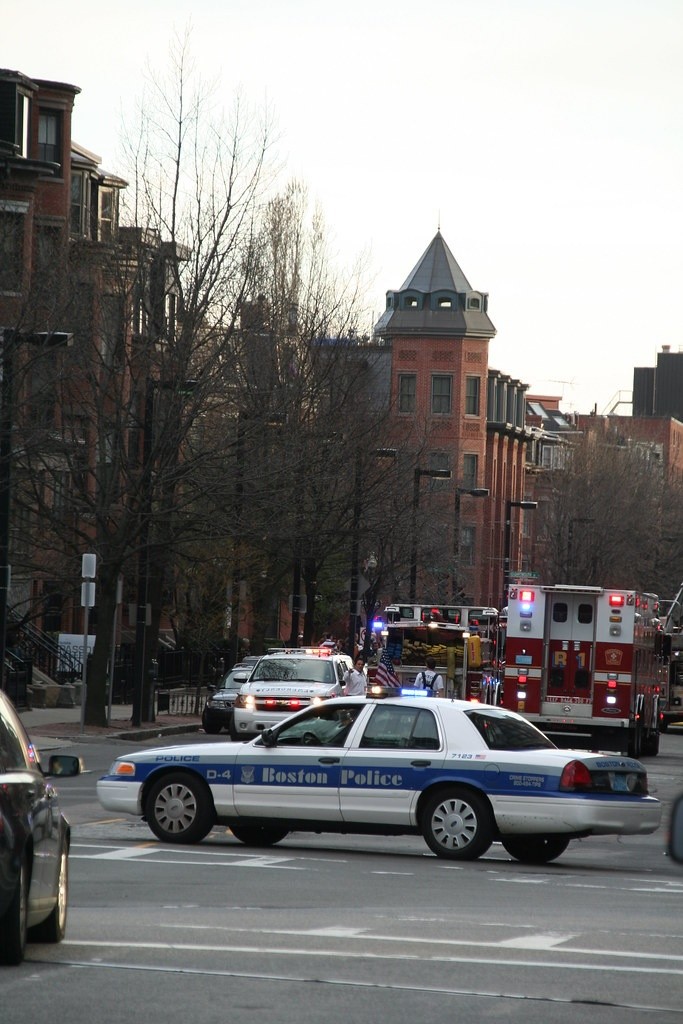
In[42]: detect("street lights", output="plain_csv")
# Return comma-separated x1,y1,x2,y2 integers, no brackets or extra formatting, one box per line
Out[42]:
291,430,343,647
230,408,288,661
567,517,596,584
654,535,678,594
349,447,398,656
410,468,452,604
0,328,75,689
446,487,489,604
131,376,201,724
504,499,538,605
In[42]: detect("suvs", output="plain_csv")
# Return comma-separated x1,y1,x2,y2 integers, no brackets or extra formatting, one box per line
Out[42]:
229,647,353,738
203,655,262,735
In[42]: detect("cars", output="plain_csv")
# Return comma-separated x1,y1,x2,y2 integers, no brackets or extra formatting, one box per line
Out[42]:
0,691,83,965
96,686,662,865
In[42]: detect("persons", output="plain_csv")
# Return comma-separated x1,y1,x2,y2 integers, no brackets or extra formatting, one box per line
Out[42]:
321,634,337,649
413,657,444,697
341,658,367,719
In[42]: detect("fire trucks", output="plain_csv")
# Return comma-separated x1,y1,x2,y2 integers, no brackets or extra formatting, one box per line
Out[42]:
504,583,683,757
372,604,508,705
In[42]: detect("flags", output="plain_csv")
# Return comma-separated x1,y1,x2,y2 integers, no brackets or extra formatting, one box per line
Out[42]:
373,647,401,688
